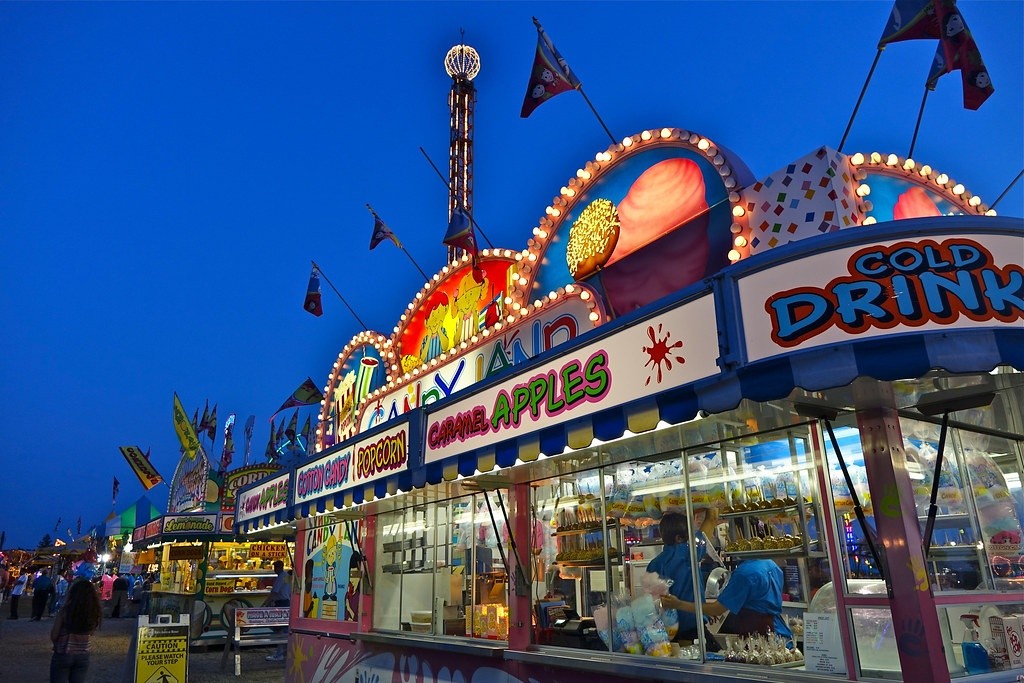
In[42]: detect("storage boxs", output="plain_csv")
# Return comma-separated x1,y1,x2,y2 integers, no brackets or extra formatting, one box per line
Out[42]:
409,611,432,634
466,604,509,641
382,536,424,552
382,559,424,572
989,613,1023,669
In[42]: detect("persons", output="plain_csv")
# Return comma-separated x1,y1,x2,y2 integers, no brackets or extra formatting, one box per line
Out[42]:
646,511,723,653
49,580,103,683
660,517,794,649
261,560,292,661
0,564,160,623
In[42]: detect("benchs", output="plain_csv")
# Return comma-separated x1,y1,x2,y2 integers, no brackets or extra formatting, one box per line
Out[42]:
219,606,289,674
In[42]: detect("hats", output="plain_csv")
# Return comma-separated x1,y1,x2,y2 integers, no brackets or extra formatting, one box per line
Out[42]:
219,555,228,562
272,560,284,566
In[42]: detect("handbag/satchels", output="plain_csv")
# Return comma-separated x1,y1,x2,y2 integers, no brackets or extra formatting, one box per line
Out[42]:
40,587,50,596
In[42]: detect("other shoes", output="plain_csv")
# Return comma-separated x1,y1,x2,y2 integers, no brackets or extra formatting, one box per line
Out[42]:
7,616,18,619
28,616,39,622
265,653,284,661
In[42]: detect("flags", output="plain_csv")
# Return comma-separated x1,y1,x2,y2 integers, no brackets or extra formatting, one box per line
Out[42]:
112,476,120,505
55,517,61,531
77,516,81,534
